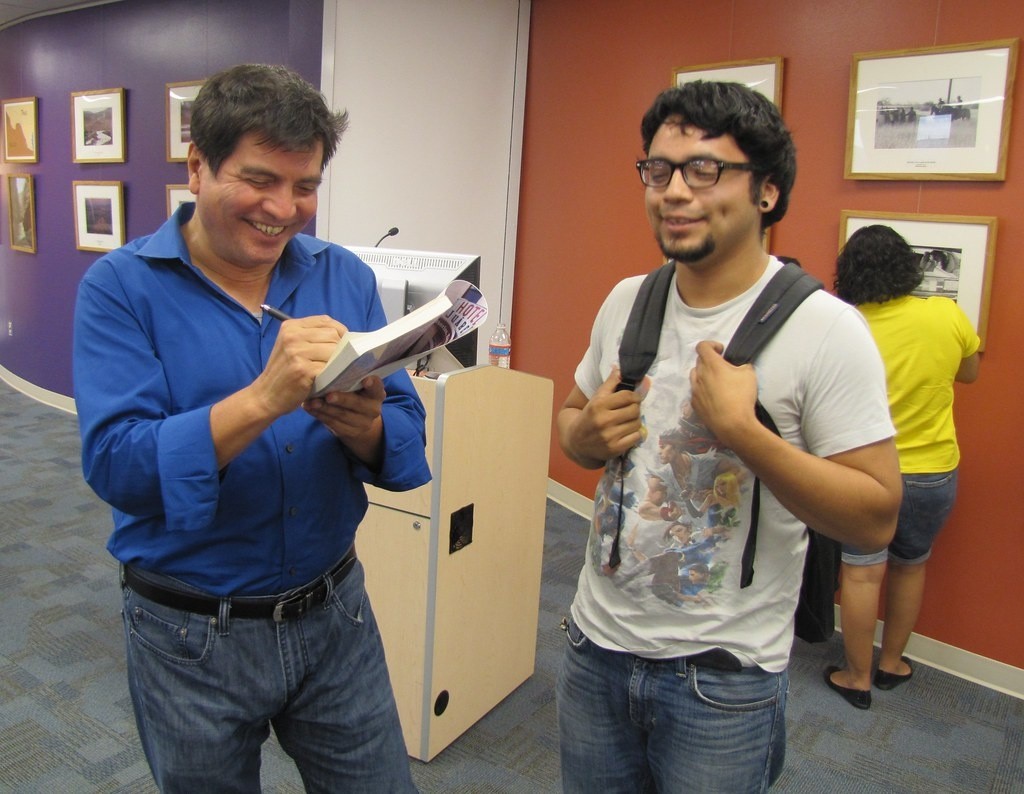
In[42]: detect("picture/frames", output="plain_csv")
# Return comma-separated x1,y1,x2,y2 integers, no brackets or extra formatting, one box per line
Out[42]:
670,55,783,117
842,37,1019,181
165,81,205,162
72,181,125,253
165,184,197,220
70,88,128,163
5,173,37,253
1,96,40,163
837,209,998,351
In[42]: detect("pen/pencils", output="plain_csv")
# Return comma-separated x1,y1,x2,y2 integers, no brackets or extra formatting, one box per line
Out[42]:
260,304,291,321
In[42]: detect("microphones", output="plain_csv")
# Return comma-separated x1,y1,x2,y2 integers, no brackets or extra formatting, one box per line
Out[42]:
375,227,399,247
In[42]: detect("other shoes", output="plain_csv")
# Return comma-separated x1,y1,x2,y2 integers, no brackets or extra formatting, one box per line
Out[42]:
823,665,871,710
876,656,914,690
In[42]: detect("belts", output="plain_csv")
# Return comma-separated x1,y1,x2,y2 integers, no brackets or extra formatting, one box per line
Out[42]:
690,647,741,673
123,543,359,619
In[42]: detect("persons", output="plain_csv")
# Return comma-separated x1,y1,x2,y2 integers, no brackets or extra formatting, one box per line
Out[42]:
822,226,979,710
74,64,435,794
883,95,962,125
559,78,903,794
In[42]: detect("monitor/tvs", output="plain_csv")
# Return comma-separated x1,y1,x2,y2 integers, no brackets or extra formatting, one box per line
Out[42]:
339,246,481,372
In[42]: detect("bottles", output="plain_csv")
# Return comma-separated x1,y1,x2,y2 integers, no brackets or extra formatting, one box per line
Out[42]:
489,323,511,369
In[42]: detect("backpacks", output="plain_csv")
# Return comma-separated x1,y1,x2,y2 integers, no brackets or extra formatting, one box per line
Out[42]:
606,255,847,642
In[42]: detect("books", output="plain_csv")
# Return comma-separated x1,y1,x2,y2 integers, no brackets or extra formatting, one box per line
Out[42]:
309,280,488,400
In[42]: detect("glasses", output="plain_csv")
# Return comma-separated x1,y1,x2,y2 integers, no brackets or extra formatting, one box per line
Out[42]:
635,157,755,192
